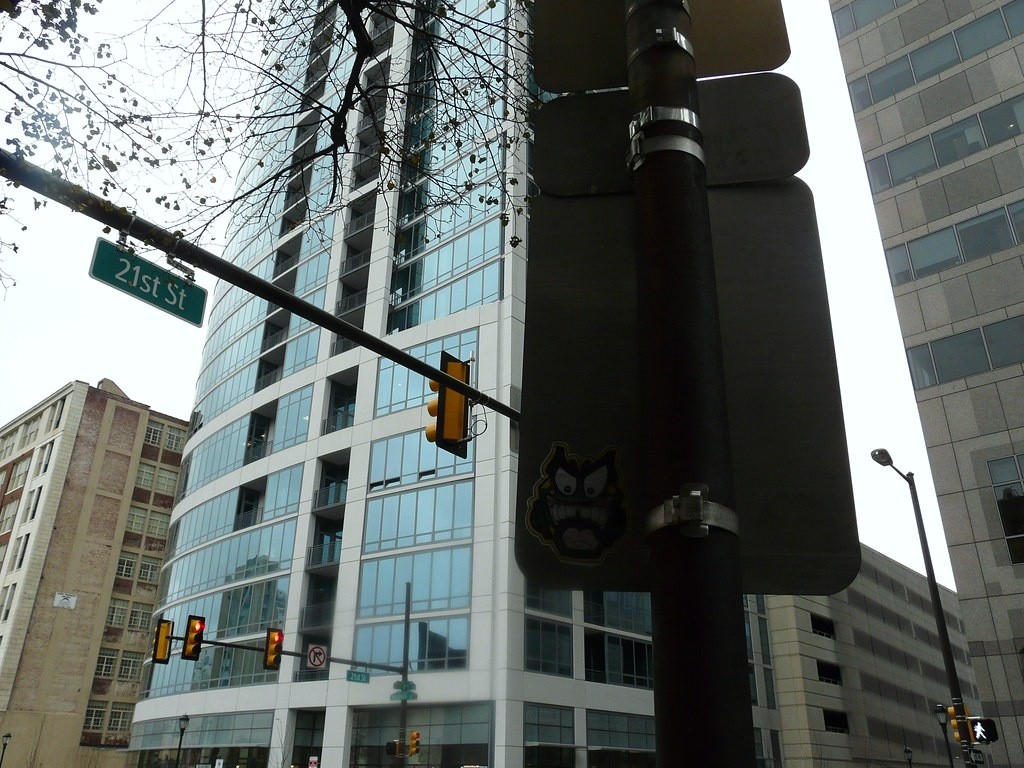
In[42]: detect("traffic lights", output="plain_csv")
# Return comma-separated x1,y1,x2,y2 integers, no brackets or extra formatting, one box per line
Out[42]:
425,350,472,459
262,627,285,671
182,614,206,662
966,716,998,743
146,618,174,664
947,706,961,742
385,740,397,756
410,731,422,757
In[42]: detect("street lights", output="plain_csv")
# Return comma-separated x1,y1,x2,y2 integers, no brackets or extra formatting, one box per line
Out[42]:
870,446,978,768
934,702,958,768
905,747,914,767
174,713,190,768
0,731,13,767
